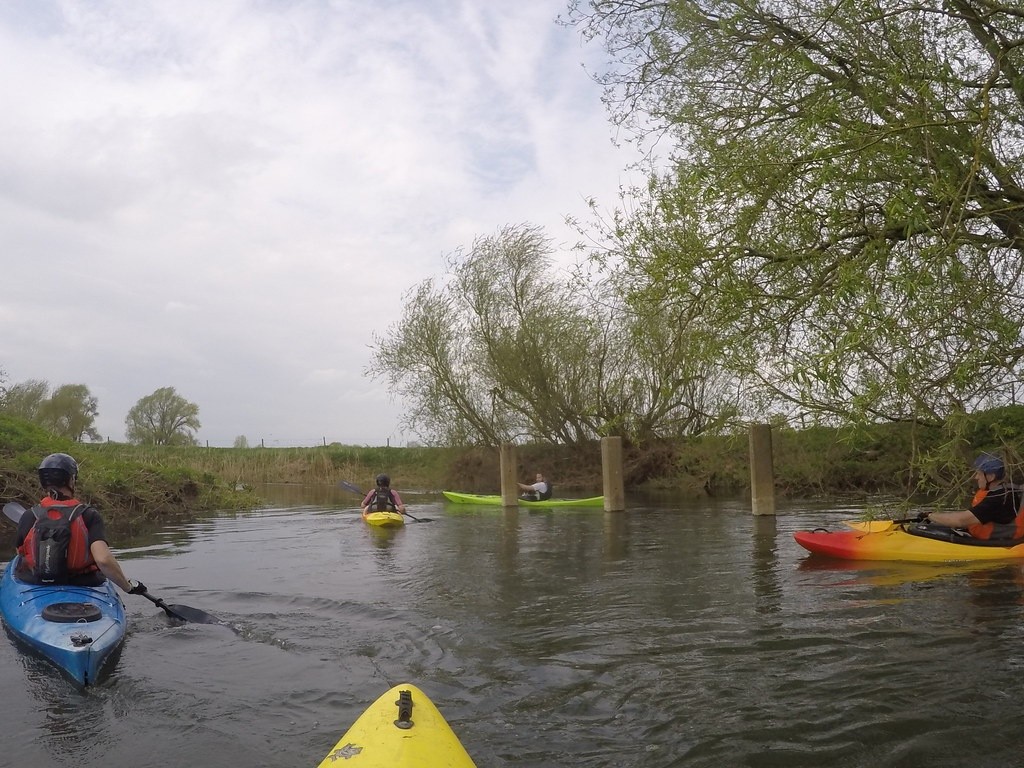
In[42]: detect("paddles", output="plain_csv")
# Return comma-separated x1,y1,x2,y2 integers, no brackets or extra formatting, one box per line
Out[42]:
841,517,920,533
2,501,222,625
337,479,433,523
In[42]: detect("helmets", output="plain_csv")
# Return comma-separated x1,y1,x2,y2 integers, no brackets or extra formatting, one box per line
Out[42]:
376,473,390,487
39,453,80,480
972,452,1005,472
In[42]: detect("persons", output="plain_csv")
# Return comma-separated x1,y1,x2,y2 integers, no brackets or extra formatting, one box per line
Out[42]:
916,452,1021,530
15,453,149,596
517,473,552,502
361,473,407,515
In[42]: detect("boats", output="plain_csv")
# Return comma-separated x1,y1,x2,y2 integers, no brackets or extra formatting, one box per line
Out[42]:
362,493,405,527
441,489,604,508
0,554,129,689
791,510,1024,563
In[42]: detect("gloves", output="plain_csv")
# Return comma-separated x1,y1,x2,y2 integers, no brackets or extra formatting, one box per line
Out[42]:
917,510,932,524
126,578,148,595
